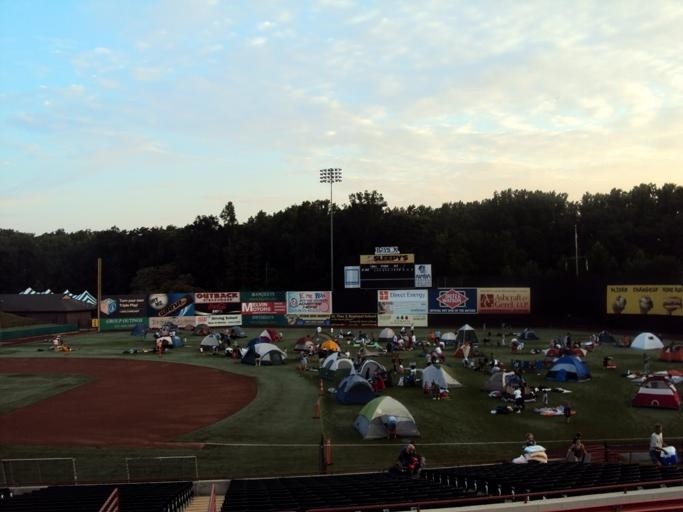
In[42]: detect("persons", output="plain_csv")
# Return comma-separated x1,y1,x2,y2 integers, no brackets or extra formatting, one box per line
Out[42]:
647,423,670,469
47,334,72,355
379,415,398,442
387,440,428,477
571,431,584,445
565,438,589,465
416,265,431,288
490,381,575,426
521,432,537,453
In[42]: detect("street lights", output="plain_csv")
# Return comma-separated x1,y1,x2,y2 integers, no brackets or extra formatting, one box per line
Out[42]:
319,167,343,291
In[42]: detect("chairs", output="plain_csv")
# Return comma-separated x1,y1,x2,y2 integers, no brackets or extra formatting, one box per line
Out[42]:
0,458,682,512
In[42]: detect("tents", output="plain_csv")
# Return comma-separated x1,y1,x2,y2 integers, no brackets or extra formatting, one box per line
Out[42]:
124,320,683,410
351,395,421,441
